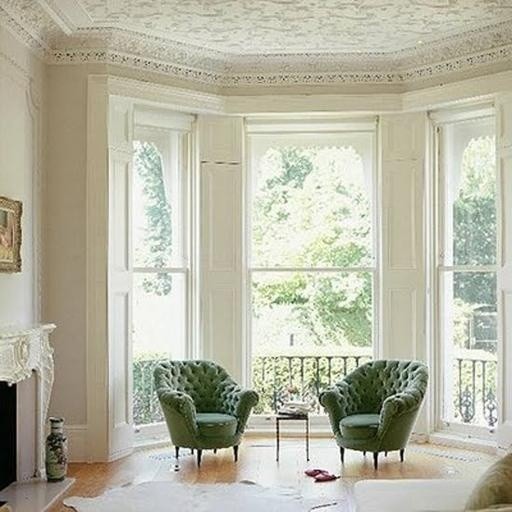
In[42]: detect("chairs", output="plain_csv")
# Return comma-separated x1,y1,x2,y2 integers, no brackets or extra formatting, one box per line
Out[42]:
319,359,429,469
154,359,259,468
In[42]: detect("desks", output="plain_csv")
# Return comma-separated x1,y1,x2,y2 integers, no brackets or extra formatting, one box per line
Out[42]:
275,406,309,462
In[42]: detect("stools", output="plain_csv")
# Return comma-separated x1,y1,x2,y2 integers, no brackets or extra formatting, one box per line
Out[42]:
352,478,468,512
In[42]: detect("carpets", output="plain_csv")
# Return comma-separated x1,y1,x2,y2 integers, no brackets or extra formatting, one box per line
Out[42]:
63,479,338,512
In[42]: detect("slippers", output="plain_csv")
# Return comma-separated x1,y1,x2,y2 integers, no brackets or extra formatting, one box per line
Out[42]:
314,474,340,481
305,469,328,477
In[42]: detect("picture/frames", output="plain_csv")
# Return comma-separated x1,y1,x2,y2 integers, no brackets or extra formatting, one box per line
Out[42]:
0,196,23,273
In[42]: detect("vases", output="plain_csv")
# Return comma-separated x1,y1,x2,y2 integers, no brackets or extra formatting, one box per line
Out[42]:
45,416,68,482
